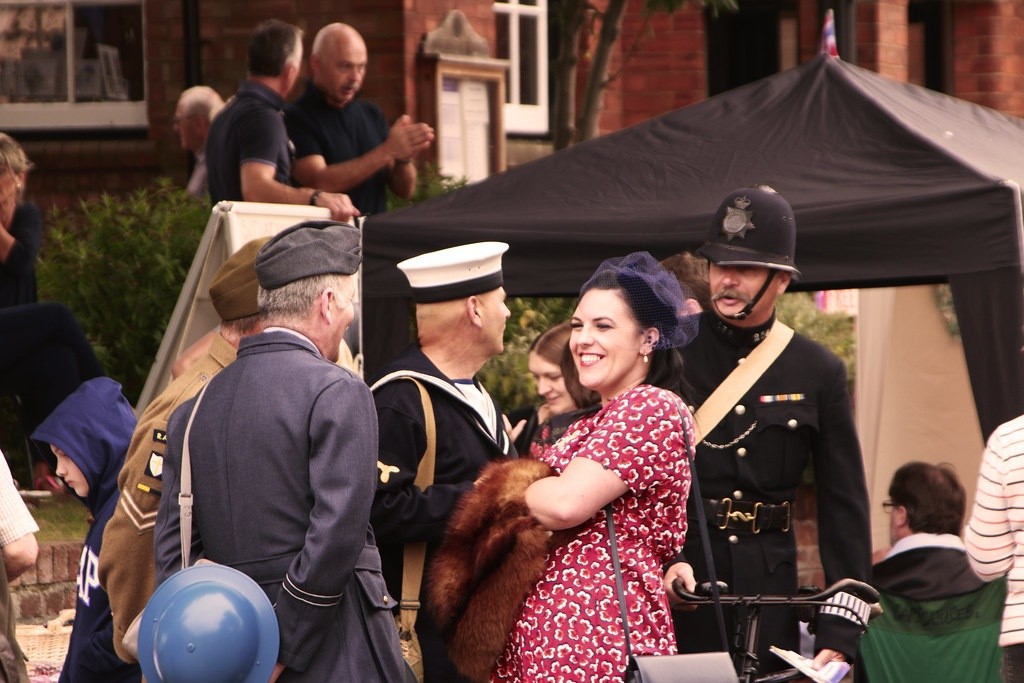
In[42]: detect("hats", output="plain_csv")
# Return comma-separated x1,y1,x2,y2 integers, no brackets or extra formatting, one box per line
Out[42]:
209,235,284,321
395,241,515,302
253,221,370,279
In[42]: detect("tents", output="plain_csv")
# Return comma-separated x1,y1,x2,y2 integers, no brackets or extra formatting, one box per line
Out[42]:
356,7,1024,449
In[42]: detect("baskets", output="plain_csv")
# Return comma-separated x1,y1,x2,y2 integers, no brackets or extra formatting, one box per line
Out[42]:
13,621,73,672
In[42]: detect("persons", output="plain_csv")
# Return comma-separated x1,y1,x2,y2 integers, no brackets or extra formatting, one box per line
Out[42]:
0,182,1024,683
169,18,435,223
0,129,104,465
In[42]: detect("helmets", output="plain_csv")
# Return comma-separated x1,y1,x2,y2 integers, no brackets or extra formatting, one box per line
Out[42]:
697,184,803,276
136,561,281,683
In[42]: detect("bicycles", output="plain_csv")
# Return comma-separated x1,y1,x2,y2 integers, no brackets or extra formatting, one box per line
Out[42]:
672,577,882,683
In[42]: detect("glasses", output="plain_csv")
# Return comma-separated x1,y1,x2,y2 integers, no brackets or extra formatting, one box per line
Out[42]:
172,112,200,126
882,499,896,516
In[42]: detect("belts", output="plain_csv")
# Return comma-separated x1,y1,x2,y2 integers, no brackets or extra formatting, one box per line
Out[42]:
682,496,799,538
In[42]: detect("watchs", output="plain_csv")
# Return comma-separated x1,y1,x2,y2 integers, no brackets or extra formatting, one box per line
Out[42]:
308,189,323,207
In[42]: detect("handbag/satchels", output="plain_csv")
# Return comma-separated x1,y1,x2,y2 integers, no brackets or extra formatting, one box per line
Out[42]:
388,619,428,683
621,649,739,683
